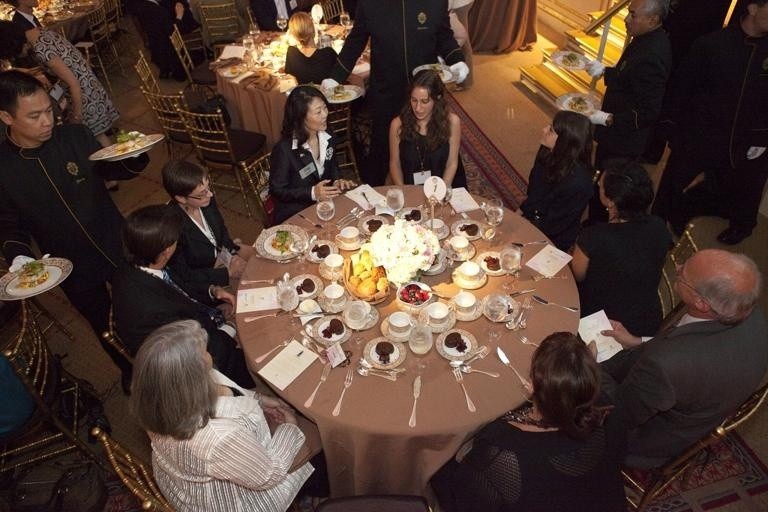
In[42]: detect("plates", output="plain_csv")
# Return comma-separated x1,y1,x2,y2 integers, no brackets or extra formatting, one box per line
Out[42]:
412,63,459,85
357,215,388,236
325,84,364,104
335,238,366,250
87,133,166,162
55,10,74,21
312,315,352,346
319,262,343,280
224,70,236,78
363,337,406,369
443,240,477,261
452,266,486,290
450,219,484,241
550,50,592,69
380,318,412,342
437,224,450,239
447,295,483,321
401,211,423,224
419,310,456,333
317,290,349,313
481,292,519,323
477,251,507,277
305,240,339,263
342,306,380,330
555,93,602,115
256,224,309,261
0,257,75,301
290,274,324,301
436,329,477,361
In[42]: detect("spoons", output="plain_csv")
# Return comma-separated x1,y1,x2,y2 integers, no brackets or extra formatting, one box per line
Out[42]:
296,212,324,230
356,365,406,382
302,337,326,365
520,316,529,329
459,363,500,377
505,301,521,330
530,274,562,281
336,206,364,228
532,295,578,313
255,254,291,264
449,346,485,367
480,201,490,222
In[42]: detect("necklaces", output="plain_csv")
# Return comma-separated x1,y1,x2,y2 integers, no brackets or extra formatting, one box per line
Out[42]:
501,406,549,431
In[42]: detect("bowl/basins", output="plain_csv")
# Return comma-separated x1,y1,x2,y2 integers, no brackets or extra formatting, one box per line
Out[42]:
396,281,434,308
299,299,317,315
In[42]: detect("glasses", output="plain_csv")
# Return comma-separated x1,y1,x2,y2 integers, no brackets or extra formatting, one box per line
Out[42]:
670,269,703,298
184,182,213,201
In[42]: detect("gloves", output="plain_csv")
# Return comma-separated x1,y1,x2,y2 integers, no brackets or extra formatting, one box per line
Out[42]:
449,61,469,85
587,60,605,80
589,110,614,128
320,78,339,93
9,254,35,274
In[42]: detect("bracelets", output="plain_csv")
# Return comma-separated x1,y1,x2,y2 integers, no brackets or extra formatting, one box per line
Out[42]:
70,114,82,120
255,392,263,406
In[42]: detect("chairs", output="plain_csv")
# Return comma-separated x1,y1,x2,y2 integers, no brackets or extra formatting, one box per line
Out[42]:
653,223,701,337
317,0,343,25
201,3,241,59
173,104,267,219
171,24,218,89
0,299,102,480
101,1,126,54
134,50,206,111
314,495,431,512
102,281,136,368
240,152,275,228
141,86,197,161
247,7,258,32
117,1,123,18
92,426,175,511
326,106,362,188
592,168,600,184
74,4,127,100
620,384,768,512
0,255,76,341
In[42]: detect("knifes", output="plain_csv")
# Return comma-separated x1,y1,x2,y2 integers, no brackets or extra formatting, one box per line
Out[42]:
510,287,536,298
408,374,421,427
293,311,332,317
496,346,530,389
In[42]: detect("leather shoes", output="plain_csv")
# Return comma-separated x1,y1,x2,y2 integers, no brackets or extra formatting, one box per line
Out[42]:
717,224,752,245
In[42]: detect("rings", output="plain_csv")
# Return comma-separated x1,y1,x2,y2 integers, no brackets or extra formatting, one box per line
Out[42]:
326,195,330,200
341,180,345,183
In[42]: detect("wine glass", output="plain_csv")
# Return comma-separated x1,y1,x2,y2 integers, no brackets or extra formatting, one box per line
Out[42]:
499,244,521,289
248,22,261,46
339,13,350,38
408,323,433,368
483,198,505,244
289,229,310,264
243,37,255,56
437,183,453,220
386,186,405,216
276,279,299,329
276,15,288,37
480,219,496,250
316,194,335,233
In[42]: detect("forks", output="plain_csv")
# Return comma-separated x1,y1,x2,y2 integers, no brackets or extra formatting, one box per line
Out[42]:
467,349,491,366
300,330,329,356
516,295,531,325
241,277,280,286
255,334,296,363
517,334,539,348
360,191,374,210
452,367,476,413
332,369,354,417
304,363,332,408
244,310,289,323
511,239,550,247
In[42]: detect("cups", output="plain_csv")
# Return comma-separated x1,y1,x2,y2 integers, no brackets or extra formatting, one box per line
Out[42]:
444,236,468,253
424,218,445,234
335,226,361,243
454,288,476,313
460,259,480,281
427,302,453,324
324,254,343,273
324,284,345,307
346,300,374,326
320,35,332,47
388,312,410,332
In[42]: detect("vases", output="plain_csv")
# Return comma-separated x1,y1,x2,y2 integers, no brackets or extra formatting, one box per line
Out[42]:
382,267,419,290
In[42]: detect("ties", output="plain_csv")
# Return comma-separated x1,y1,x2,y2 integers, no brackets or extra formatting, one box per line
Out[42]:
163,270,226,327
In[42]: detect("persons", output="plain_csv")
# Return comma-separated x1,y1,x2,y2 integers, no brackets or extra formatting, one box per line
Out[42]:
430,331,626,512
448,0,473,92
385,70,466,189
4,1,43,67
0,69,150,397
269,85,358,221
160,159,254,283
514,111,594,248
569,159,672,337
113,204,253,387
582,0,767,244
118,1,207,83
588,249,767,476
340,0,469,188
250,0,358,86
128,319,322,512
0,20,120,192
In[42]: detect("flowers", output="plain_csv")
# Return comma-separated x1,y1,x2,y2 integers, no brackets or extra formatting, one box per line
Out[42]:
368,218,441,285
